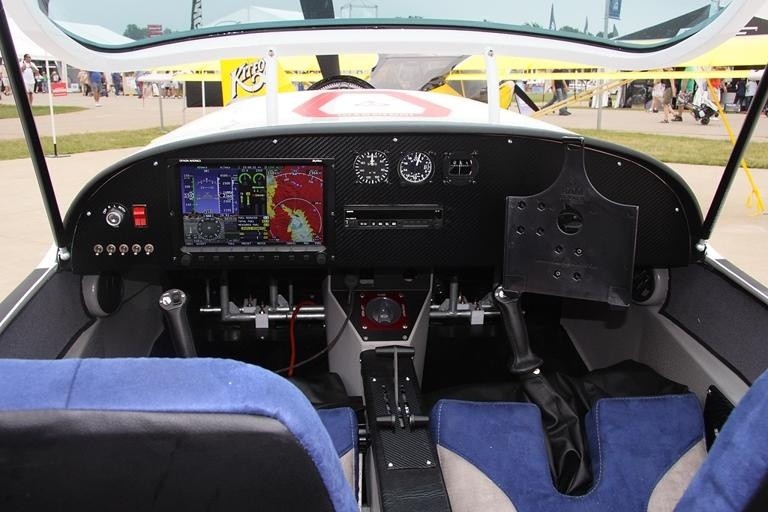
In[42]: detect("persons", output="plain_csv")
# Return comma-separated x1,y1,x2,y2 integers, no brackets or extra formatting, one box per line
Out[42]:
544,70,573,115
0,49,182,108
632,66,768,126
535,68,569,116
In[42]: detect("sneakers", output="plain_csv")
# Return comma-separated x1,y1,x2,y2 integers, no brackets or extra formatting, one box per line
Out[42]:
559,111,571,115
661,115,682,123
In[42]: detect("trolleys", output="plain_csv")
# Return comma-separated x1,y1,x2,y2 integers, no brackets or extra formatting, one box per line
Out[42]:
675,92,720,125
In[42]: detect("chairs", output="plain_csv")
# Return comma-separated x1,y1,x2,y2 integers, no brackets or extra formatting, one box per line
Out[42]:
1,354,363,512
429,369,768,512
